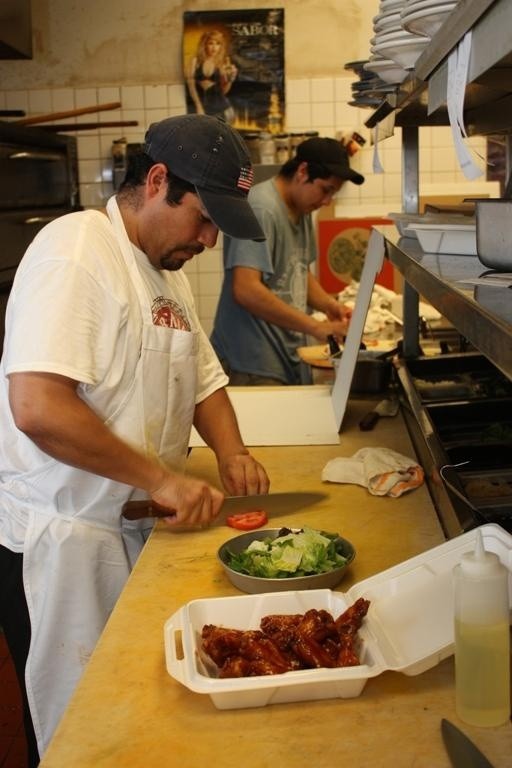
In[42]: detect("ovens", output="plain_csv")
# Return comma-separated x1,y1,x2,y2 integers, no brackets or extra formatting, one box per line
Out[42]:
0,120,85,291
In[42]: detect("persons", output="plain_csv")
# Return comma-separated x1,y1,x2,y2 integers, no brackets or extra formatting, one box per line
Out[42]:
210,138,365,386
187,29,238,128
0,113,269,768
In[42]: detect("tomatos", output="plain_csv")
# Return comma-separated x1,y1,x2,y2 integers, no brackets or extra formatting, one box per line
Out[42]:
225,510,268,529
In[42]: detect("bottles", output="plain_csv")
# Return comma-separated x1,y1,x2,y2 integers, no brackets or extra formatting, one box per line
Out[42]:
112,139,128,168
246,131,320,165
452,527,512,728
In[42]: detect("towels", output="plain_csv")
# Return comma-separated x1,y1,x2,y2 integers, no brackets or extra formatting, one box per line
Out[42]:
322,446,425,499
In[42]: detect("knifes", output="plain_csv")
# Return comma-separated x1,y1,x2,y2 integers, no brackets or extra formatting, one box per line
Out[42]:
122,493,327,526
360,392,400,432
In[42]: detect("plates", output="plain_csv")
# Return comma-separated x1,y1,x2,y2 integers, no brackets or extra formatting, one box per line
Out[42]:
217,527,357,594
344,0,460,110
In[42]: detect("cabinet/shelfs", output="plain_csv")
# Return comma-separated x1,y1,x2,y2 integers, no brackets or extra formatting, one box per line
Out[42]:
366,0,512,541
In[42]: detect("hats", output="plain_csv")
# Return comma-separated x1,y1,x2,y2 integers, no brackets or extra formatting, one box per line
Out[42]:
297,137,364,185
142,114,267,243
